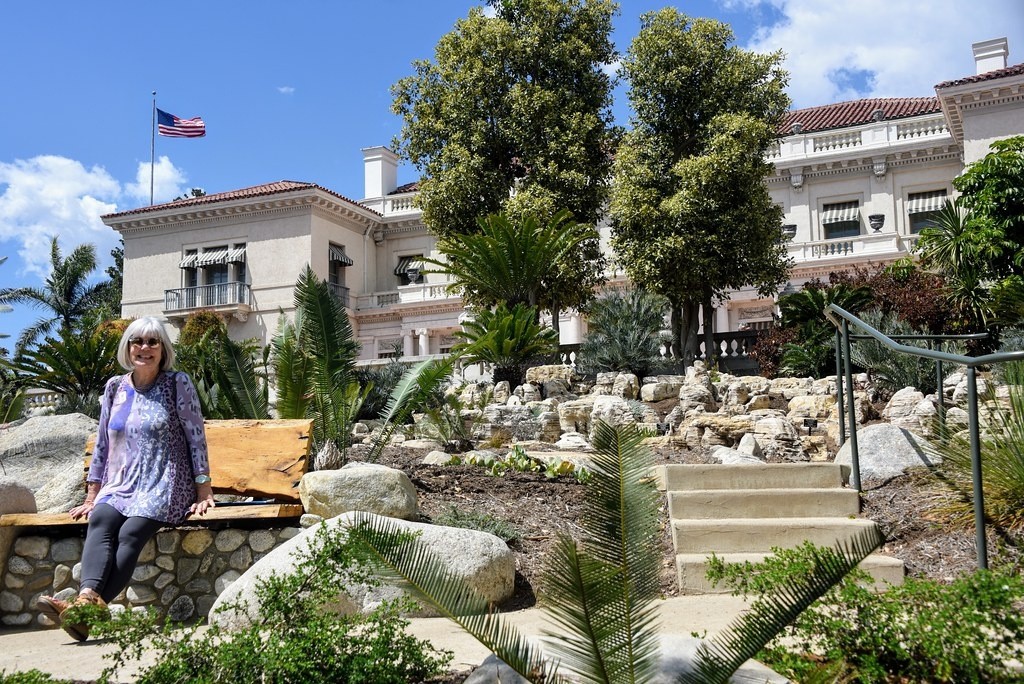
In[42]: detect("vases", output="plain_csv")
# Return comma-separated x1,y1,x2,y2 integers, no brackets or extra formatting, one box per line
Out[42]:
792,121,803,134
867,213,887,235
874,108,883,123
779,223,800,245
406,268,420,284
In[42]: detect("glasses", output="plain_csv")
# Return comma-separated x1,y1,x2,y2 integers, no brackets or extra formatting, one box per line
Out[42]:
129,337,161,347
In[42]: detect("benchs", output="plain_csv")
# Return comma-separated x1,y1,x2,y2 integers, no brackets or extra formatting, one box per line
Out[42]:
0,411,317,529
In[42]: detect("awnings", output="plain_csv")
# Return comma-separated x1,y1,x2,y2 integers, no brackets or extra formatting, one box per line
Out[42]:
328,244,354,267
178,245,246,271
393,257,423,277
907,189,946,214
820,201,860,225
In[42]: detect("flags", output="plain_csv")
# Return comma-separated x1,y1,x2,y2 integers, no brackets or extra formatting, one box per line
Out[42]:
156,107,207,138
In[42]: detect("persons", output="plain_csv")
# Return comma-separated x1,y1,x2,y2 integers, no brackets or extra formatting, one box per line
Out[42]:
37,317,215,641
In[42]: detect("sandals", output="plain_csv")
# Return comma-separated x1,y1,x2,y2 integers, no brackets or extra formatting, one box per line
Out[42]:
38,594,93,641
59,593,112,626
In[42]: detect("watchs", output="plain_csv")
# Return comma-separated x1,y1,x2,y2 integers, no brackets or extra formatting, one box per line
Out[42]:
195,475,211,484
